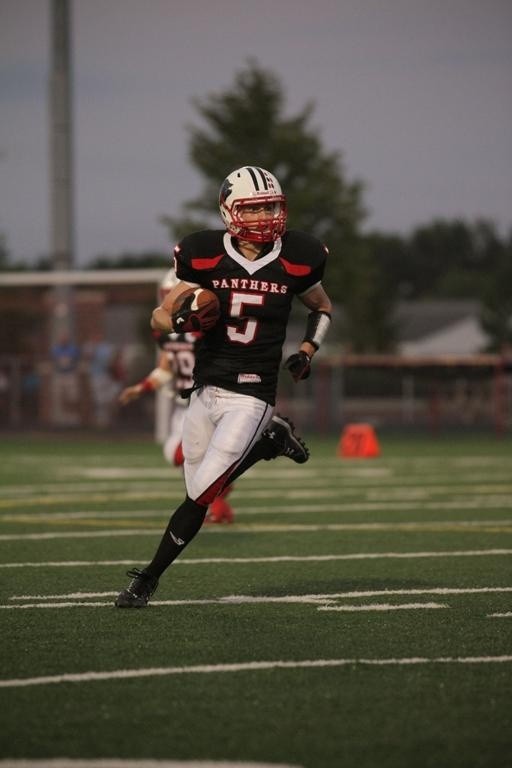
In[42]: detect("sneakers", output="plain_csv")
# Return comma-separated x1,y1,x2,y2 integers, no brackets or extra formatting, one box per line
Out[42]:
116,571,158,608
261,413,310,464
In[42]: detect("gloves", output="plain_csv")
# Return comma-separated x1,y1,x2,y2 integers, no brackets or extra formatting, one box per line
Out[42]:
171,293,220,333
284,350,311,384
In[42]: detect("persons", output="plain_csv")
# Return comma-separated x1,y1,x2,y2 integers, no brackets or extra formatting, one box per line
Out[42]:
1,329,155,436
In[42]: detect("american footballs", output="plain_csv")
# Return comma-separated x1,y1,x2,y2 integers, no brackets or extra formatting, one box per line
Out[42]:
172,288,220,317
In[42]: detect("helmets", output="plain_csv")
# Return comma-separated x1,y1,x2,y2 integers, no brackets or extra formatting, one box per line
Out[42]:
218,166,288,242
